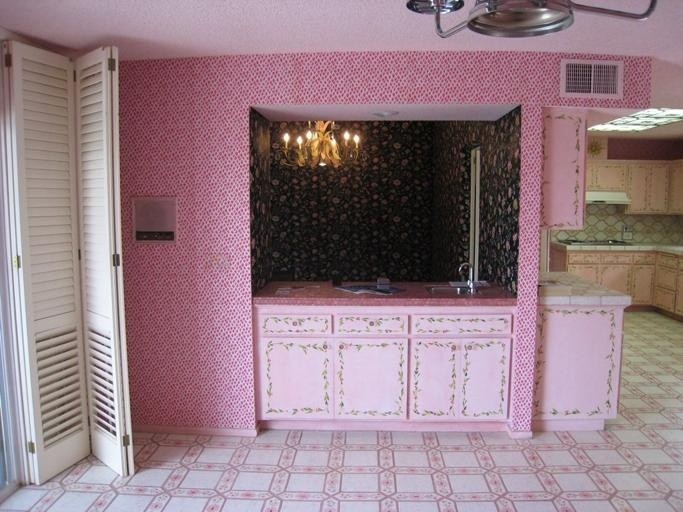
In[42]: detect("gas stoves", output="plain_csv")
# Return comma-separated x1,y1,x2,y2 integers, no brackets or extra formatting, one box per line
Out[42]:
559,238,632,246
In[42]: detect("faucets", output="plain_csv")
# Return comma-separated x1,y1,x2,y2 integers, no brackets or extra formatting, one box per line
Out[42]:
459,262,474,292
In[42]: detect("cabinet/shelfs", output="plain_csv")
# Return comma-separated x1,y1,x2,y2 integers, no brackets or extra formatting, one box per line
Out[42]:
251,303,518,433
550,248,600,288
599,250,657,312
656,252,683,321
583,159,683,215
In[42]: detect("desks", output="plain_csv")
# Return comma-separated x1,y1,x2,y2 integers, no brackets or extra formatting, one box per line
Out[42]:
531,268,633,434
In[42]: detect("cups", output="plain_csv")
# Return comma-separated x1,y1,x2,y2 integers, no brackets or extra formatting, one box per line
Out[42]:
332,274,342,287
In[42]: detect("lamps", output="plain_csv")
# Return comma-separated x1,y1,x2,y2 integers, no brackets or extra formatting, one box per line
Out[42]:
273,118,364,170
406,0,659,40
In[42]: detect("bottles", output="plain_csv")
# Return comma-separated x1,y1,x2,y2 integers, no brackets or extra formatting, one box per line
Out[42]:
376,273,391,291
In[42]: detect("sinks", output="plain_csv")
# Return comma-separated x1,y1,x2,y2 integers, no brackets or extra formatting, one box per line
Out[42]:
425,286,460,296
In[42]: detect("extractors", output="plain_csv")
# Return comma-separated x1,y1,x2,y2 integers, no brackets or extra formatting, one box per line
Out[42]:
585,190,631,206
